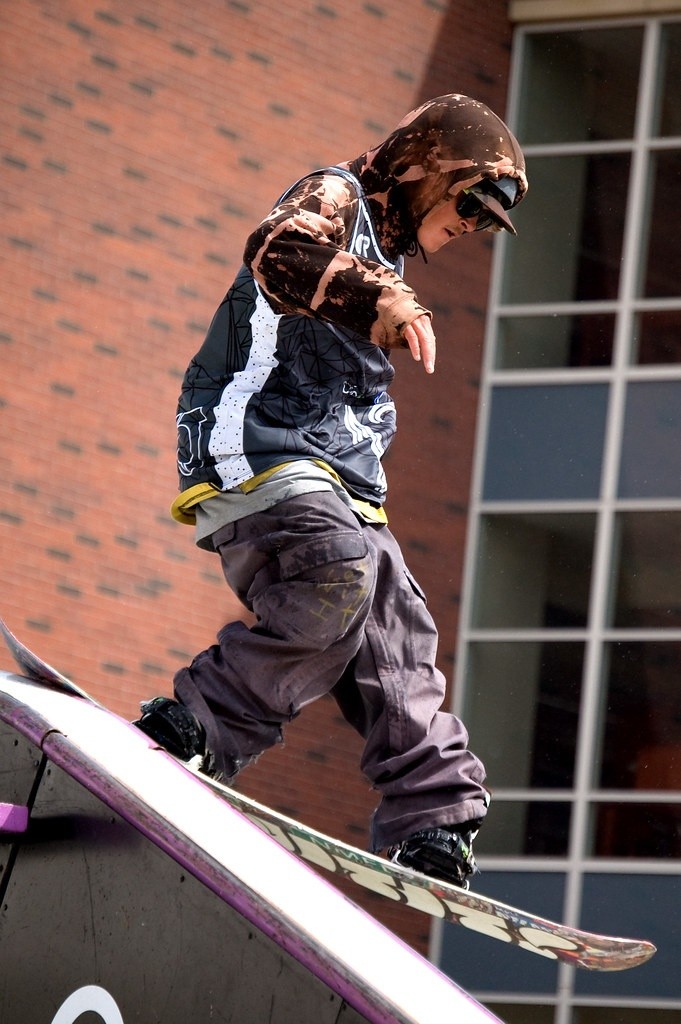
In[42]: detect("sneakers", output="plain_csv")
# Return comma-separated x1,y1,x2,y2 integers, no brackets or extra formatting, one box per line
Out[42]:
387,829,478,890
130,697,225,780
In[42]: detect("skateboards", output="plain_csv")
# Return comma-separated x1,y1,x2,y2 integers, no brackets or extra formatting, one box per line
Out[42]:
0,616,659,977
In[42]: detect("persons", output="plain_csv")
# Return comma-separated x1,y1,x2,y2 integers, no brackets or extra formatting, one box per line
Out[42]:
128,94,530,891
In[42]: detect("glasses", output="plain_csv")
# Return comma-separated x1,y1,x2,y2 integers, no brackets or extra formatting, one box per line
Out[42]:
456,192,493,232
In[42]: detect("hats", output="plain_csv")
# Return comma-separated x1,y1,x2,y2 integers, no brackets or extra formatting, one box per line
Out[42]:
468,177,518,237
